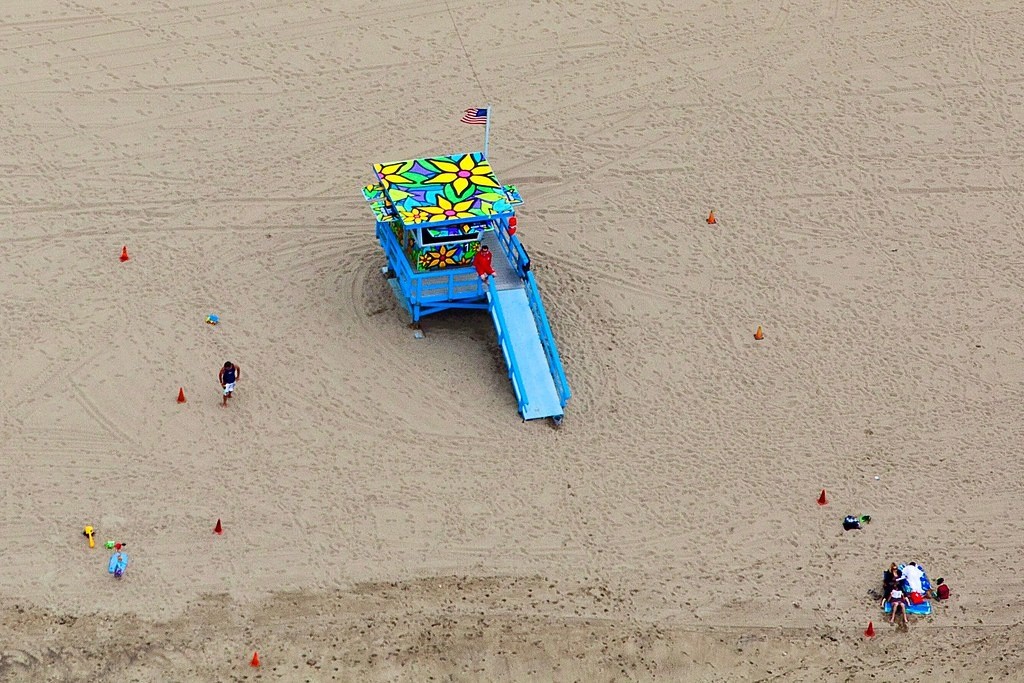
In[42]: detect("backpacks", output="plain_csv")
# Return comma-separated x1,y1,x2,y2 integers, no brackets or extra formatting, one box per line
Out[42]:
938,584,950,599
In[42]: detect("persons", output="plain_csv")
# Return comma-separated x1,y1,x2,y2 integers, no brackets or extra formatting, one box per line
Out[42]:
472,245,497,281
218,361,240,406
879,561,950,624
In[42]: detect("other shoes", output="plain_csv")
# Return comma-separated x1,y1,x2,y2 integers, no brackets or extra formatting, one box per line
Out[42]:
874,595,882,600
868,589,874,594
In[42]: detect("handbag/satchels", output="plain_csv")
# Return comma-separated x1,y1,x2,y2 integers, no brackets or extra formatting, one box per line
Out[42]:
911,592,923,604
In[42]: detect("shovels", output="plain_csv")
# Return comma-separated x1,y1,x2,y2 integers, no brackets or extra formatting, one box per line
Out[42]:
85,525,95,548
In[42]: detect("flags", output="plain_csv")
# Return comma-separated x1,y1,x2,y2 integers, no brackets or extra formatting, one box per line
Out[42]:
460,108,487,124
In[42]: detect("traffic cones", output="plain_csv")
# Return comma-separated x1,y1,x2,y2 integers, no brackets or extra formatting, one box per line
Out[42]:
249,651,261,668
864,621,877,637
118,246,129,263
176,387,188,404
212,518,224,535
706,210,716,224
754,325,766,340
816,489,829,507
